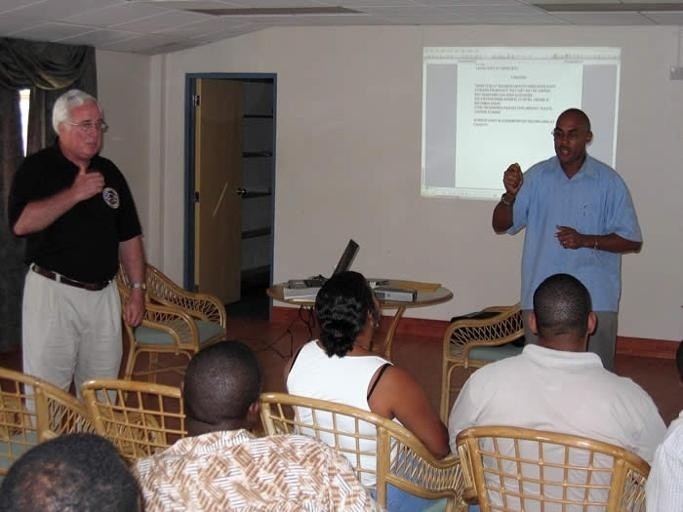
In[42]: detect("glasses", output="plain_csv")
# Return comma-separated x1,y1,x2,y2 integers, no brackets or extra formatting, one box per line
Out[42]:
65,121,110,135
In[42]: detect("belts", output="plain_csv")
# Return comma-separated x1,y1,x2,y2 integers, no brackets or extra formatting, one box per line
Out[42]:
32,264,116,290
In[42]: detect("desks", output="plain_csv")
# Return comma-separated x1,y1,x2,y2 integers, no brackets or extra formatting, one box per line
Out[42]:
261,278,450,370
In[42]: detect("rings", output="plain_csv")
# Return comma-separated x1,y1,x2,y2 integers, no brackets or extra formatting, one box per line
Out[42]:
562,240,567,245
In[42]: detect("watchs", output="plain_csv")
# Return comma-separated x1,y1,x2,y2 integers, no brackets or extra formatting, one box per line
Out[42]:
126,282,146,292
501,193,515,207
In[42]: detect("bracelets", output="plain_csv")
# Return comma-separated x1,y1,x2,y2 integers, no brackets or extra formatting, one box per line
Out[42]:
593,235,601,250
491,107,643,370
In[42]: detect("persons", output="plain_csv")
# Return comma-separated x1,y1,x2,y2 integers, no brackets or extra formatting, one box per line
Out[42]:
282,271,478,512
446,273,667,512
6,90,146,434
644,338,683,512
0,432,144,511
129,340,388,512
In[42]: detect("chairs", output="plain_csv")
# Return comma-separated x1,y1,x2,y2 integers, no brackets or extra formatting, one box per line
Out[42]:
439,296,532,420
251,388,470,511
72,375,189,463
446,423,653,511
2,365,158,483
109,259,227,389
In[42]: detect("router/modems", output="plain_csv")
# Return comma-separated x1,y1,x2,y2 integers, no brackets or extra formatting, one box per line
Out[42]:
374,287,417,302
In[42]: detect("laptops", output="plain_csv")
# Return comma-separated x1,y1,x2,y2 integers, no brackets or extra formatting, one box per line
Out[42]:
288,239,360,289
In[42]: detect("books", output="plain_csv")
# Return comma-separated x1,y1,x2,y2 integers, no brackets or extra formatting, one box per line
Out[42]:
367,277,388,285
283,278,325,303
396,282,442,291
